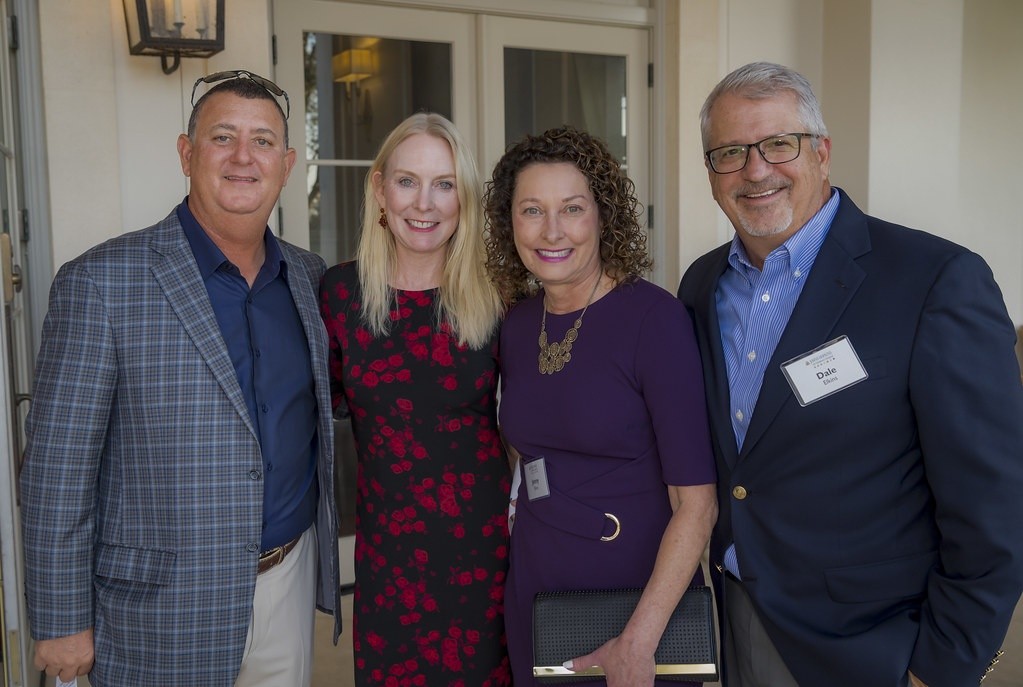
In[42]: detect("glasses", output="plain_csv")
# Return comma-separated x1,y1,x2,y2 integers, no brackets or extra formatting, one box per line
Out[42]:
706,132,825,174
190,68,290,121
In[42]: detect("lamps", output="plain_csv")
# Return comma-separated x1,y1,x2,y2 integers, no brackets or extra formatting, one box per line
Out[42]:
332,48,374,100
121,0,227,74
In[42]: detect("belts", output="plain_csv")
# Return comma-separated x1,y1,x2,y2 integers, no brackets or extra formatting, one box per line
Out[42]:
257,532,303,575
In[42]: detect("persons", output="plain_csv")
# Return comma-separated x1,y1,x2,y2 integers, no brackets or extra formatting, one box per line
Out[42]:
482,126,719,687
675,61,1022,686
19,78,350,686
314,113,518,687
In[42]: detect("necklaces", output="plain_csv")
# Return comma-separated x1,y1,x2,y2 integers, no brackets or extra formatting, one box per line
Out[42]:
537,266,603,375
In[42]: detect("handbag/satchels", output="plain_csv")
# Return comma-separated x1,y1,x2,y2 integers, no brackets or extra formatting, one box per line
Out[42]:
531,586,720,687
